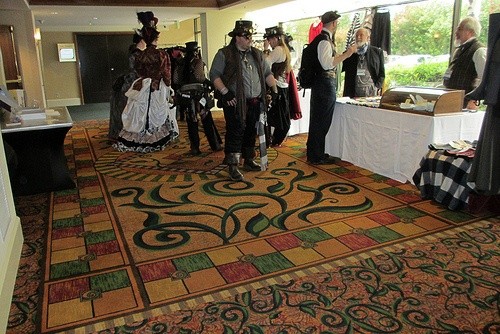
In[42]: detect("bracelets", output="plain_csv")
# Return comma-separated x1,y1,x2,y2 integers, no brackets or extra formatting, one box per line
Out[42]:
263,50,269,54
221,87,227,93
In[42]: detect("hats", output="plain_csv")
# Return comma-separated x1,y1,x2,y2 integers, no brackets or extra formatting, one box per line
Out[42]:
263,26,283,37
181,41,201,51
227,20,257,37
321,10,341,24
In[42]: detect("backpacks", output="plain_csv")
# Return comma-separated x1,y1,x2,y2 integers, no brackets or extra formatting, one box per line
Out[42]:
298,42,315,89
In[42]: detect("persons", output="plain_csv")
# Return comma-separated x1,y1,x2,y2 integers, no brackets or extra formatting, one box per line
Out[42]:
173,42,222,157
306,10,357,163
209,20,277,180
263,26,302,148
109,10,179,151
342,28,385,98
443,16,488,95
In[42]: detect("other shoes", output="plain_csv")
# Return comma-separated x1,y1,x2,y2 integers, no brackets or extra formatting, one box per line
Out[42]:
190,149,201,155
307,153,335,164
244,159,262,171
229,166,244,181
212,144,224,151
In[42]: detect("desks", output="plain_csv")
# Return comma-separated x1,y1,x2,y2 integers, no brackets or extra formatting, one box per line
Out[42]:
1,106,77,199
325,97,486,187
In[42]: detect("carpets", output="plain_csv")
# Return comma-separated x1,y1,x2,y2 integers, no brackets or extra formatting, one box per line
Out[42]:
6,111,500,334
